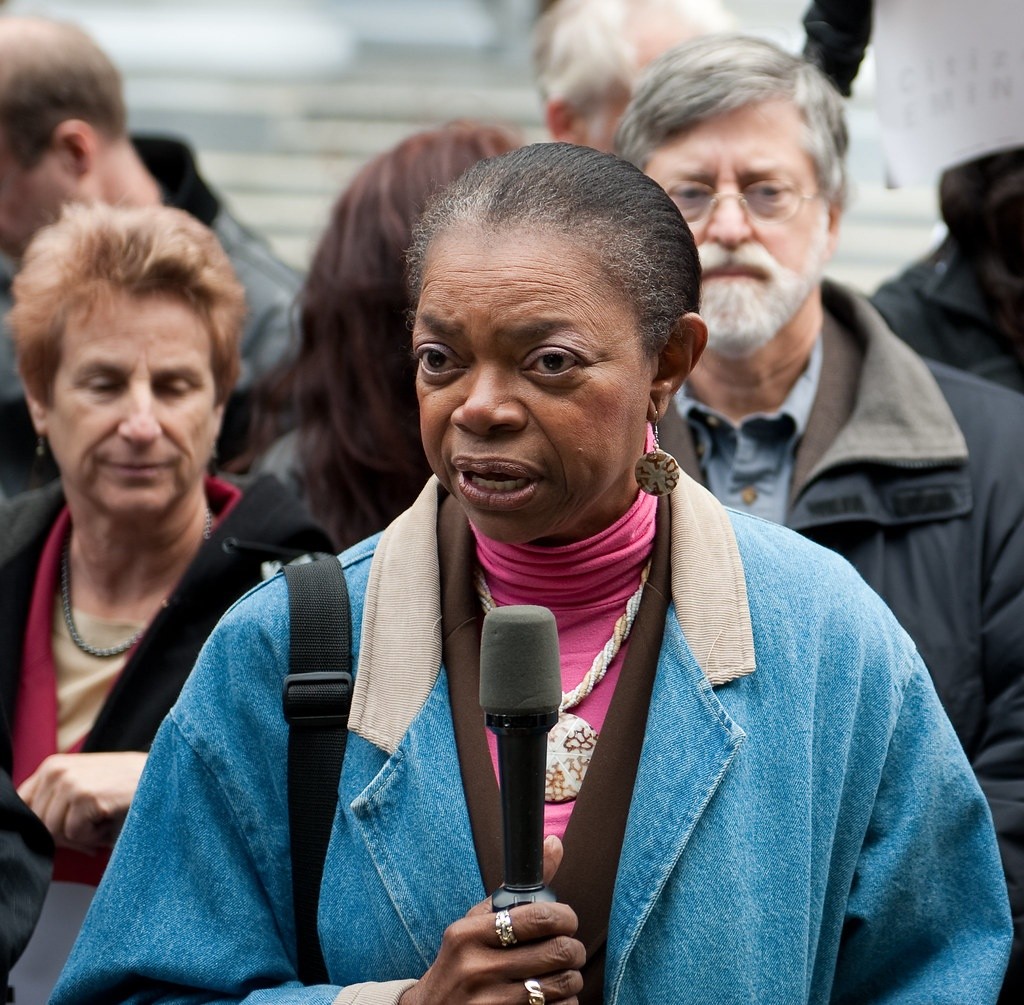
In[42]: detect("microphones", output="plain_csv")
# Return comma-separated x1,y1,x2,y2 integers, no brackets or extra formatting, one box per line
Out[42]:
480,605,561,911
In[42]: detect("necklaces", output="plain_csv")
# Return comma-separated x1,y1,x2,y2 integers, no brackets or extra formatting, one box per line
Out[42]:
475,559,652,803
61,506,214,658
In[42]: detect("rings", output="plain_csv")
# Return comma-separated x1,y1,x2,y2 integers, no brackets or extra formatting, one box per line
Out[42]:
523,978,545,1005
495,909,517,947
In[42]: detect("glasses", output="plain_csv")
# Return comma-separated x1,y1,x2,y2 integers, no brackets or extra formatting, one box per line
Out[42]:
664,177,834,227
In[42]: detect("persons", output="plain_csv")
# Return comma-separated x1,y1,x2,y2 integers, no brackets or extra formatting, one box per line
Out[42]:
0,204,341,1005
531,0,741,154
251,120,524,549
44,141,1016,1005
610,33,1024,1005
0,14,309,394
